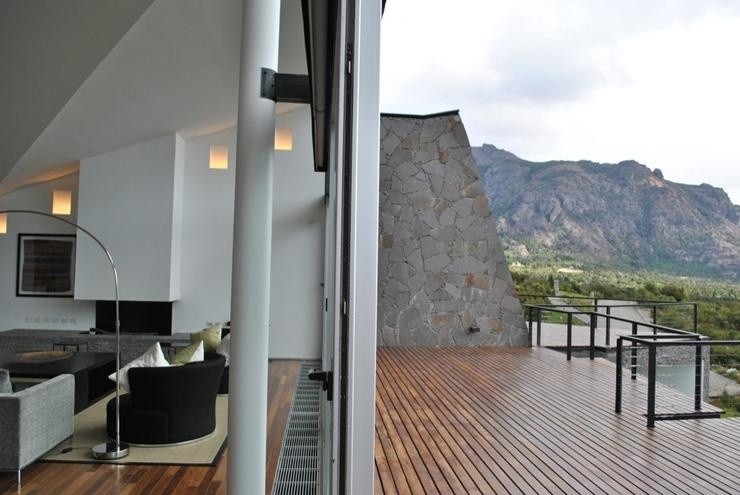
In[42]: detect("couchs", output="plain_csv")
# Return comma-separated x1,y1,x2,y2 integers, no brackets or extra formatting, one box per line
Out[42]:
106,329,230,449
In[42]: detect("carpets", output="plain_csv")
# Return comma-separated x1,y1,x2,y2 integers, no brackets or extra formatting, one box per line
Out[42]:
35,388,229,467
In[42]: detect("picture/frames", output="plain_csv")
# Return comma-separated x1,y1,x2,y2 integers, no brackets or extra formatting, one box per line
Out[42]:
16,232,76,297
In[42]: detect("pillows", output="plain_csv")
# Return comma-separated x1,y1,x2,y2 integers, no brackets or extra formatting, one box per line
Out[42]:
0,368,14,394
108,322,230,398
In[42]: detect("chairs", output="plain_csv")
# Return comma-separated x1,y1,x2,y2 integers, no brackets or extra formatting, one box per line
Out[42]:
0,368,75,484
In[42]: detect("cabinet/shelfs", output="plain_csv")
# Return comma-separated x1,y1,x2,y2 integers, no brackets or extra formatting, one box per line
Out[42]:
0,328,200,387
